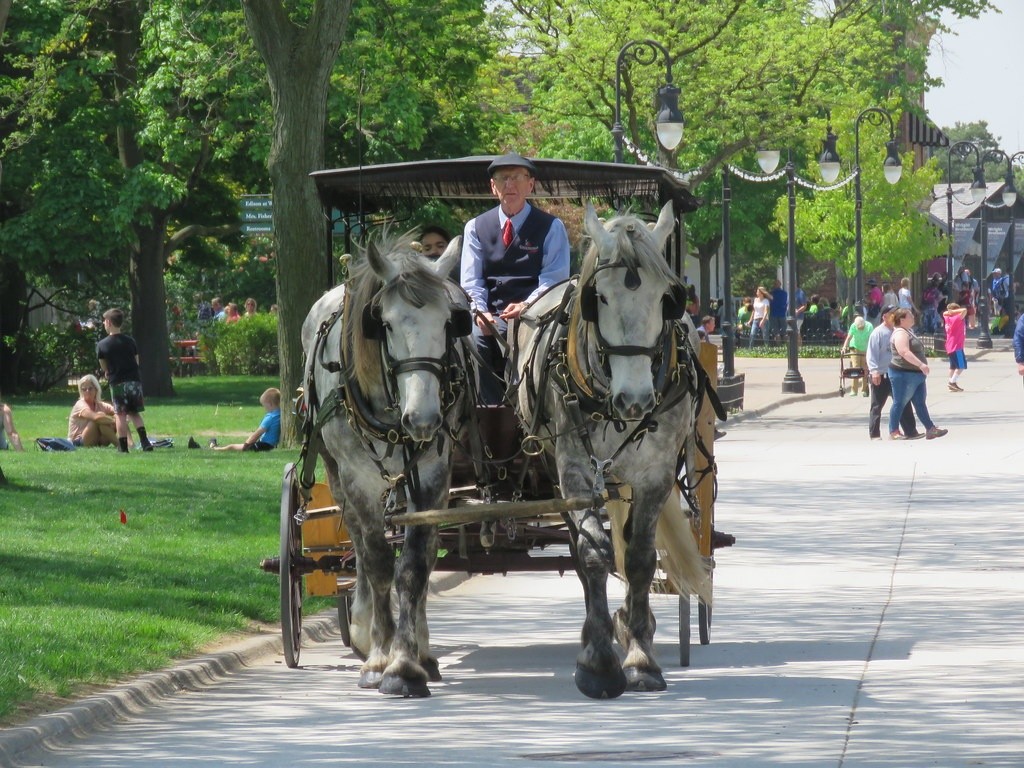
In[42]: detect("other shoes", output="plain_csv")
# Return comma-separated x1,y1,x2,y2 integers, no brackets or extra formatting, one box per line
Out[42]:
871,436,882,441
142,442,153,451
890,430,907,440
188,436,201,448
863,390,869,396
948,382,963,392
208,438,217,450
903,430,926,440
850,391,857,396
926,425,948,439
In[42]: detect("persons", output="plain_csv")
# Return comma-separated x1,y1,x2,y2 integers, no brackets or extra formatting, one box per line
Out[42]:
696,316,715,343
866,304,926,441
419,226,451,257
748,287,770,348
795,284,807,346
841,317,873,397
809,295,855,330
928,267,1009,329
864,278,920,327
738,296,753,330
887,307,949,440
686,284,699,314
194,291,258,341
0,404,23,452
764,280,787,345
943,303,968,391
1013,313,1024,376
95,309,153,453
68,374,134,448
207,387,281,452
460,153,570,404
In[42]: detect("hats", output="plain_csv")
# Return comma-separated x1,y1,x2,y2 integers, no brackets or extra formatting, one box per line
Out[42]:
488,155,536,176
880,303,897,315
865,279,878,286
992,268,1002,275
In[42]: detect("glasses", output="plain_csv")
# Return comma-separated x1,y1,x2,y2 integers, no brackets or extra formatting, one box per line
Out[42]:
81,388,96,392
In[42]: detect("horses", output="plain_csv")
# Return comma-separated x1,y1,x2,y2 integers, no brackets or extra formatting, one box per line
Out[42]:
299,234,480,701
502,194,702,700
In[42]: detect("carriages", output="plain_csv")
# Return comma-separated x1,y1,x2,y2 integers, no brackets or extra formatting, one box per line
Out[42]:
254,154,740,704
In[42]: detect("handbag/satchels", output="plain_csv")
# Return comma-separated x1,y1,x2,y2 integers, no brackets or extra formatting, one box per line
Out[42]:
36,438,75,452
867,306,879,318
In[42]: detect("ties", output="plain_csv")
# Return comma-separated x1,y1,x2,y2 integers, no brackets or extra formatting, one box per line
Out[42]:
502,220,514,248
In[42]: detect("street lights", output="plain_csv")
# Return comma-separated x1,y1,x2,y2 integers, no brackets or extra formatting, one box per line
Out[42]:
711,137,780,411
781,102,842,396
608,38,687,218
944,141,989,312
854,106,902,332
970,148,1023,351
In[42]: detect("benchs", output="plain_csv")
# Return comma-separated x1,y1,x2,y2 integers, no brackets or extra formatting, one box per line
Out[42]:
168,340,205,378
